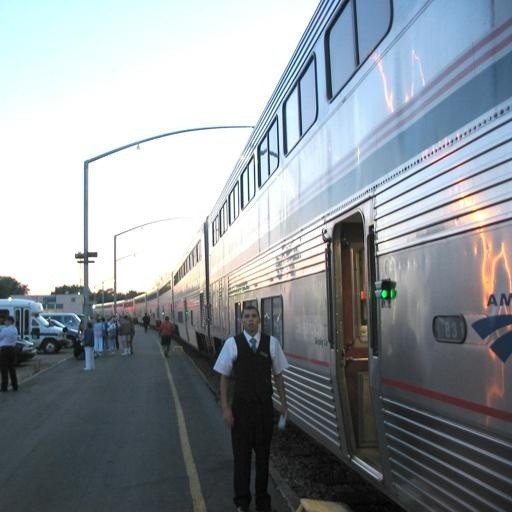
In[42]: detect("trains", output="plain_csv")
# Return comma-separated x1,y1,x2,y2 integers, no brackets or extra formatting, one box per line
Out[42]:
91,0,512,512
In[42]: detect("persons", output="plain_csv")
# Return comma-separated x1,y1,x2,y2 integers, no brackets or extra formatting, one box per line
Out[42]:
214,304,290,511
0,316,18,392
142,313,150,333
75,314,135,371
158,316,174,357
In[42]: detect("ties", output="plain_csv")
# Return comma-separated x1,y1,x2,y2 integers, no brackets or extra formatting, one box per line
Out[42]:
249,337,259,353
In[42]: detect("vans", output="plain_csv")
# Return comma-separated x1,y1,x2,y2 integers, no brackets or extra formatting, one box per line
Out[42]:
40,313,81,329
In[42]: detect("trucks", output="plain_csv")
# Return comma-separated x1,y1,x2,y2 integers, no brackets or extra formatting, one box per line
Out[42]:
0,297,67,354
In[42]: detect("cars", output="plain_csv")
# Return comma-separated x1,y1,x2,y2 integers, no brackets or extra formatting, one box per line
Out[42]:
47,319,79,348
